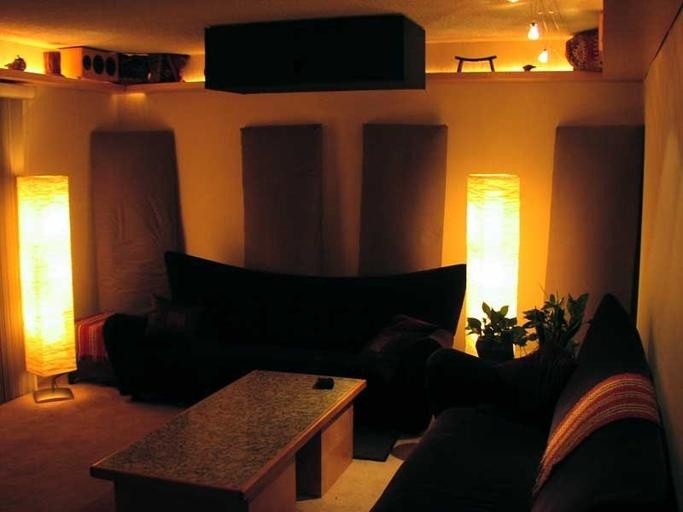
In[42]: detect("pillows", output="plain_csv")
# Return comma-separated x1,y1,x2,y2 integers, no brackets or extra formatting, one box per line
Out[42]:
147,290,213,352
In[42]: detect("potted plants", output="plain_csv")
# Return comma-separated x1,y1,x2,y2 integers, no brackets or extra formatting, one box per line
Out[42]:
461,291,589,359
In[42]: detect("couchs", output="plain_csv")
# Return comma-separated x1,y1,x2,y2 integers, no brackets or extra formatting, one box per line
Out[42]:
101,250,466,460
368,293,679,511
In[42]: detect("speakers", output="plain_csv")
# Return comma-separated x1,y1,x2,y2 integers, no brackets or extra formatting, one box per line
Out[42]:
59,47,119,82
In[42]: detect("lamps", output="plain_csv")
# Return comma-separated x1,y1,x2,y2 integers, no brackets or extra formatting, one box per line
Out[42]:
14,174,79,404
464,170,520,357
